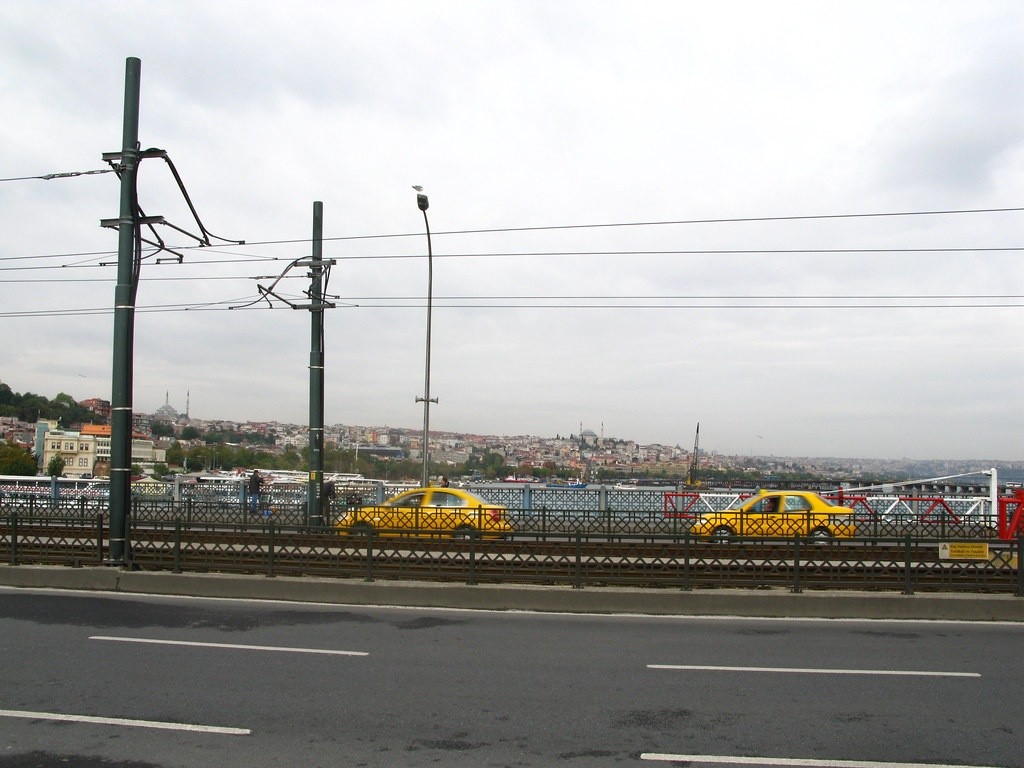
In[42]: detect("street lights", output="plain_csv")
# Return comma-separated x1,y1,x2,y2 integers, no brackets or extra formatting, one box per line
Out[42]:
409,193,443,486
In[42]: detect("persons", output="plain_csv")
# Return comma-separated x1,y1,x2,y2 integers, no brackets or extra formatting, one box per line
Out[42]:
440,478,449,489
763,502,774,511
249,470,264,514
318,478,338,526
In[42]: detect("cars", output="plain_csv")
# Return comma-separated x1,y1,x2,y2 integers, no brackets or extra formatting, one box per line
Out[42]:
690,488,854,546
335,489,513,543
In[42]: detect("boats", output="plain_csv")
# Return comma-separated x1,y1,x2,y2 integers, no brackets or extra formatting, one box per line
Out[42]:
503,469,637,492
190,458,421,503
